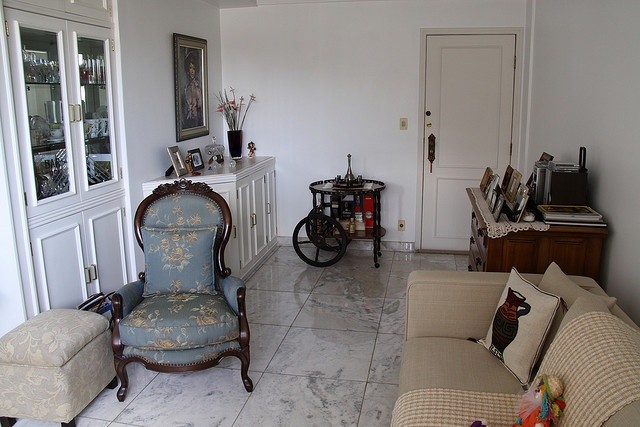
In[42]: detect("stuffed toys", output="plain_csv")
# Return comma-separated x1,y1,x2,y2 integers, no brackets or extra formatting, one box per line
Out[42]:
468,373,568,427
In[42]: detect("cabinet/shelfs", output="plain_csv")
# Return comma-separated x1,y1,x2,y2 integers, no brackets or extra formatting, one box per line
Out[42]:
465,186,608,283
141,154,280,285
292,177,386,269
0,0,134,320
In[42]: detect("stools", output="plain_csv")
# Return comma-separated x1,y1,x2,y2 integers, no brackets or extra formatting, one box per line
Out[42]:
0,307,113,424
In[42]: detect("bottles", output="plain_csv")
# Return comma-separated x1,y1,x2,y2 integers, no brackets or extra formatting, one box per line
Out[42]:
95,55,106,84
348,217,355,235
344,153,356,182
21,43,59,83
84,53,96,84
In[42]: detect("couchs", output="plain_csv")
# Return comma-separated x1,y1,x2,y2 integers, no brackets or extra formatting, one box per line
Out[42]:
392,270,639,426
108,178,254,403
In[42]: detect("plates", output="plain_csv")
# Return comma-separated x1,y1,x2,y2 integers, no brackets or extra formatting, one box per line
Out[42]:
29,115,51,139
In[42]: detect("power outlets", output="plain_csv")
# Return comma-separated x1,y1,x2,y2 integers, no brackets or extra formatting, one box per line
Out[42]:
398,219,405,230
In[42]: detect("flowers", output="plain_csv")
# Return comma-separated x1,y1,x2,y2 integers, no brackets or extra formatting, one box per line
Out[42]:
213,85,257,130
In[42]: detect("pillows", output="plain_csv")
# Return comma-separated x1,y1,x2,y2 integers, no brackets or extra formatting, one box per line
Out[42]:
532,294,639,425
537,260,618,362
140,226,219,298
478,264,562,385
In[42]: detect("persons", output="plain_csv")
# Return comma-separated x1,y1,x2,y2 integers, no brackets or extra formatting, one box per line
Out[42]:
181,47,203,129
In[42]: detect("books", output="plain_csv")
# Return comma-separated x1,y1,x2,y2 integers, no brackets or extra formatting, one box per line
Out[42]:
537,204,609,228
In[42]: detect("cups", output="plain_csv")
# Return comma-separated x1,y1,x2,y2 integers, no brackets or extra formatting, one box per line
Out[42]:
99,117,109,136
85,118,104,138
44,99,64,125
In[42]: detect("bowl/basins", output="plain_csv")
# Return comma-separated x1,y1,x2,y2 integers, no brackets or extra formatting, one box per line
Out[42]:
49,128,63,138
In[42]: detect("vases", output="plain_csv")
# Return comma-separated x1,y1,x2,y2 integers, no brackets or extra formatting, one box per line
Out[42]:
226,129,244,159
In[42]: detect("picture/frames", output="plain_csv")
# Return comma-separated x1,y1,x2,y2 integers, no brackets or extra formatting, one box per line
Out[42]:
488,188,498,213
506,168,522,202
514,194,530,222
187,147,205,170
500,164,515,193
166,145,188,177
526,150,554,189
486,174,500,205
511,182,529,206
482,174,494,199
478,166,493,191
492,189,506,223
172,32,210,142
